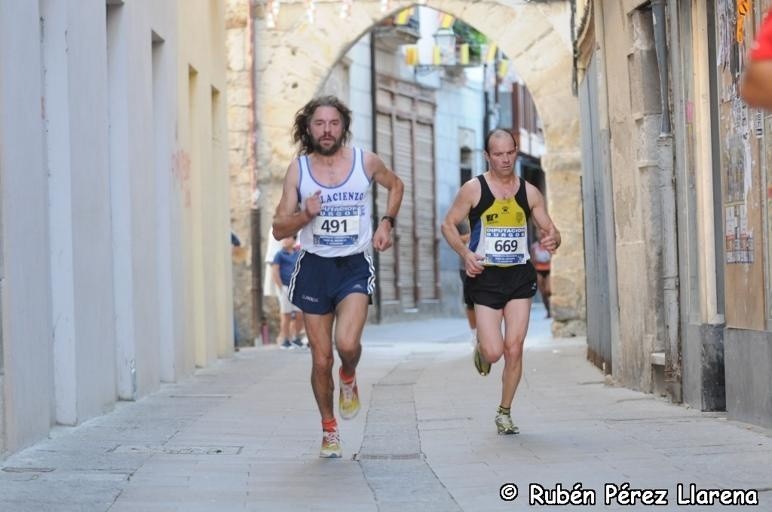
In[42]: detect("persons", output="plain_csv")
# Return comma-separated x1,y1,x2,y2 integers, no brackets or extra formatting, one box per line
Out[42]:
458,216,478,329
272,232,306,351
733,4,772,122
529,228,551,324
271,95,406,460
442,127,560,437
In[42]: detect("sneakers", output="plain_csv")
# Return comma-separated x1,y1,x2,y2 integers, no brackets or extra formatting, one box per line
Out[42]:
474,335,490,376
293,338,306,347
320,425,342,457
339,366,359,419
281,341,295,350
496,409,519,434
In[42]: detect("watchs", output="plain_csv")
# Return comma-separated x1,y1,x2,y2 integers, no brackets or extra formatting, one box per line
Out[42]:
381,216,394,228
